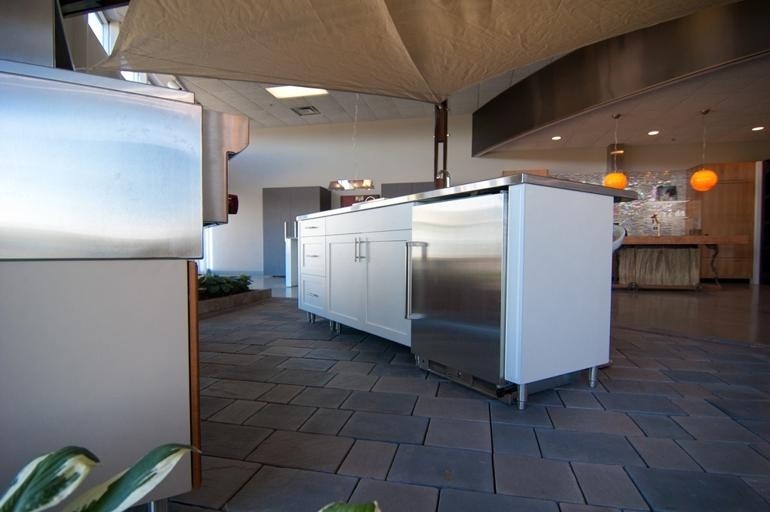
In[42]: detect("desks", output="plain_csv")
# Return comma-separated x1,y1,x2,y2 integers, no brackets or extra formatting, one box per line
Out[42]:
617,236,753,291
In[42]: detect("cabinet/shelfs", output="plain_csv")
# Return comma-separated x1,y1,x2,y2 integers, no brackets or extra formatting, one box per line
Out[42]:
326,201,414,348
401,189,506,398
299,216,326,312
1,59,249,512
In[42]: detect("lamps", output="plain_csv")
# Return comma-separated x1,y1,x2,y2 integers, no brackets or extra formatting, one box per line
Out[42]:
603,107,717,191
